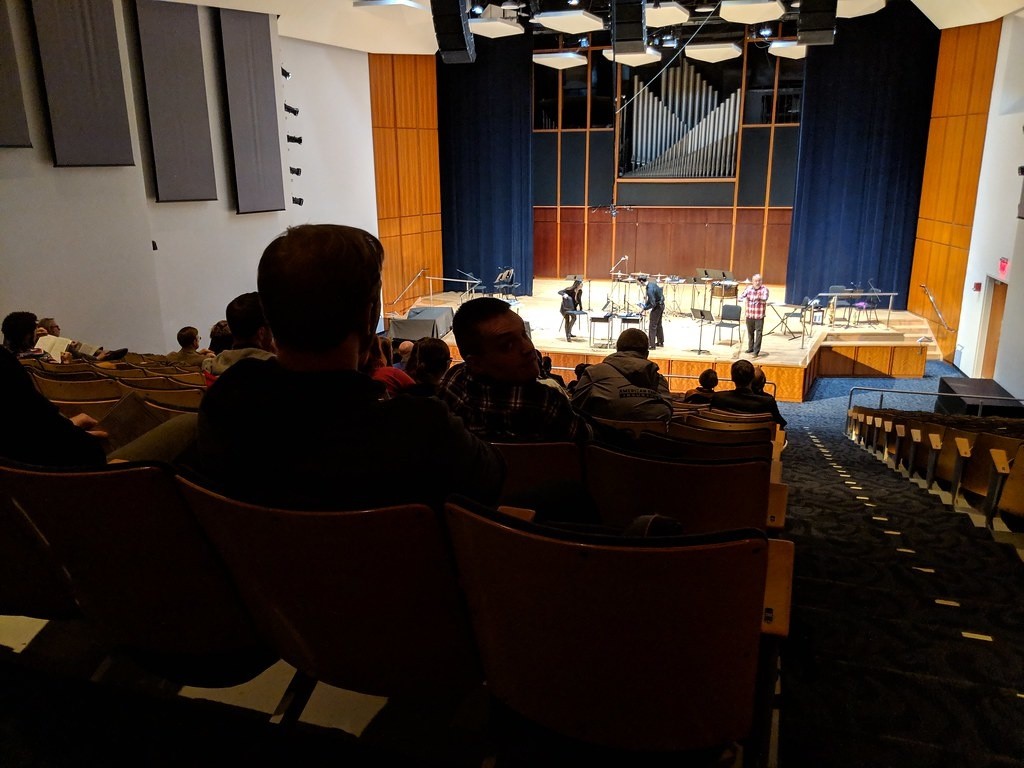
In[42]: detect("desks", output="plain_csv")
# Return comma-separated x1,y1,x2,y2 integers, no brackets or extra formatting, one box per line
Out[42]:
762,302,809,340
589,315,613,349
818,293,898,331
620,315,644,333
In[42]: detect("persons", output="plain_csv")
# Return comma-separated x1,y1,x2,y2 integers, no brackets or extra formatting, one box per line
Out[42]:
737,274,769,358
637,275,666,349
557,280,583,342
440,298,594,447
359,336,508,416
176,292,278,377
2,312,197,468
566,330,787,432
197,225,507,513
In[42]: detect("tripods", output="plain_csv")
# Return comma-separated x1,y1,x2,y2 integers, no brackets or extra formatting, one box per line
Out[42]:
841,289,864,329
663,268,735,355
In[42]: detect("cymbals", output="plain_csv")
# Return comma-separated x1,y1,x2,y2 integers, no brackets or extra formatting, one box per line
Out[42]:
621,279,637,281
651,275,666,276
739,281,752,285
631,273,650,276
610,272,627,276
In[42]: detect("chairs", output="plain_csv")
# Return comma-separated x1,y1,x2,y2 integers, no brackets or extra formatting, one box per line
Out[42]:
0,353,791,768
558,296,589,331
781,290,819,335
856,289,880,324
468,272,516,299
847,405,1024,531
826,286,851,327
713,305,741,346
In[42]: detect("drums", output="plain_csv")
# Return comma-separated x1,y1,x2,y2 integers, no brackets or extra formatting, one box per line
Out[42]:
710,281,737,299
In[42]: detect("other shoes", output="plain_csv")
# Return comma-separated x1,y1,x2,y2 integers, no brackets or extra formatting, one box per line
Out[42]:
567,338,572,342
752,352,759,358
102,347,129,361
570,334,577,337
656,343,664,347
745,349,753,353
649,345,657,350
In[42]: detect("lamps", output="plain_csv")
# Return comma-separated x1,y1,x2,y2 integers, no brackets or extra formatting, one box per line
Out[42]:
471,0,800,47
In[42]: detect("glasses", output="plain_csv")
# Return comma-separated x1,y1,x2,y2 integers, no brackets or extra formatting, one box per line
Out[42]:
51,325,59,330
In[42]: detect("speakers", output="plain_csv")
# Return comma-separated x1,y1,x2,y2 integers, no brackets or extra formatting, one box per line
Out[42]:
611,0,646,54
430,0,478,63
798,0,838,45
